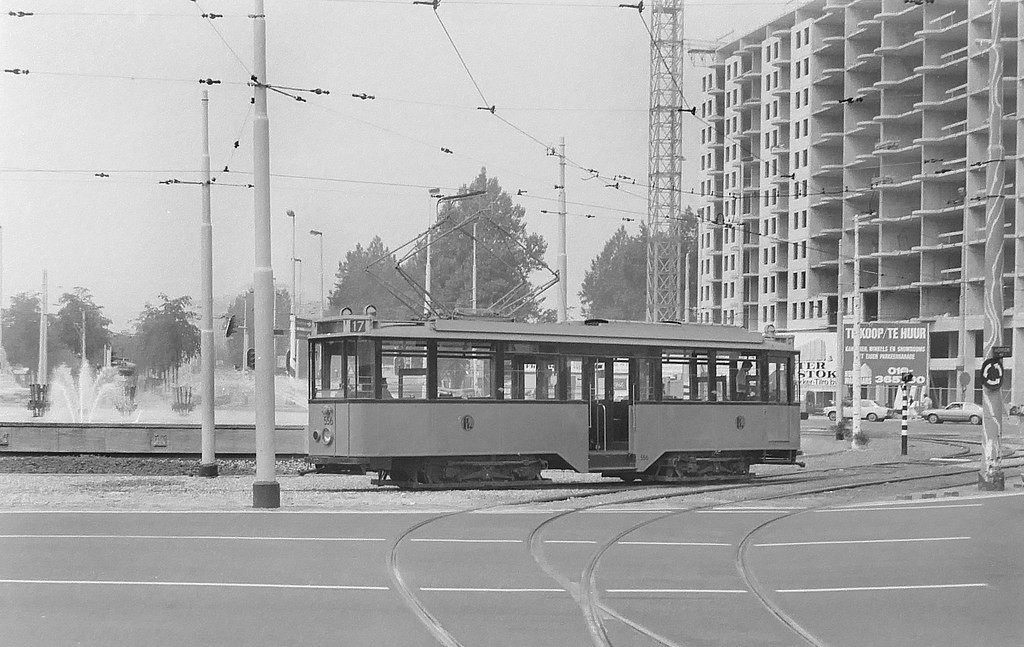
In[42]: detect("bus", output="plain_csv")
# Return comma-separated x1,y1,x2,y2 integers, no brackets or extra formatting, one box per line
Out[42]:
298,306,804,489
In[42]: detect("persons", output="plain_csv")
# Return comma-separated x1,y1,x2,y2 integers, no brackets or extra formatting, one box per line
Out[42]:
736,361,753,400
921,394,932,410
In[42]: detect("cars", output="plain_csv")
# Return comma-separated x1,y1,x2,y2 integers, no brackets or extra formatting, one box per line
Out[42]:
921,402,986,425
824,399,894,422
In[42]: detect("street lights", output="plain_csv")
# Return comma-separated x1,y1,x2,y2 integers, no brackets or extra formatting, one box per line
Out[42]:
286,208,297,382
421,187,441,400
310,230,323,318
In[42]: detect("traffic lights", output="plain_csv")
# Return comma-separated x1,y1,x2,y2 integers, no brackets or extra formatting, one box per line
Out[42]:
247,347,255,370
